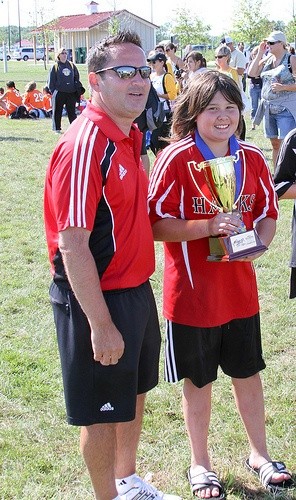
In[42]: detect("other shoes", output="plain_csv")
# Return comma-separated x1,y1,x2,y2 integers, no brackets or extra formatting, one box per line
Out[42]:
56,129,61,134
252,126,255,130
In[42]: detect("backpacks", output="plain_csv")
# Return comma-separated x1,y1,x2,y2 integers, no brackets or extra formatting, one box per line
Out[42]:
17,106,29,118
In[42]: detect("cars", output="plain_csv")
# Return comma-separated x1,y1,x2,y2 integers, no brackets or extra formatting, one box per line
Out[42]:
0,52,10,61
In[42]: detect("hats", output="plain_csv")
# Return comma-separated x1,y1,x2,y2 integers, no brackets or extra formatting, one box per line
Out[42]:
220,36,233,44
147,52,167,62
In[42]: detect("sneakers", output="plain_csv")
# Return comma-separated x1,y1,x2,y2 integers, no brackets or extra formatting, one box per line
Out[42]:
118,470,184,500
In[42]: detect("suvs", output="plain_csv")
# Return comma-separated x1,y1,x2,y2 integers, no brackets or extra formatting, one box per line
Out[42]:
14,47,46,61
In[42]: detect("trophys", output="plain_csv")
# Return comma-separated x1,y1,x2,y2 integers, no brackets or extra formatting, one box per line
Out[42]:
187,149,268,262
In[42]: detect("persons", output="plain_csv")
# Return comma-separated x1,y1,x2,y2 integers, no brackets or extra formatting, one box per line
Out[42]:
47,49,79,134
274,129,296,298
23,81,52,112
146,69,292,500
43,33,182,500
246,31,296,178
211,37,262,131
0,81,23,117
133,43,206,179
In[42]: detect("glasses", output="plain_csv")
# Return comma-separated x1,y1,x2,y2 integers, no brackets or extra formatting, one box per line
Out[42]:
267,41,281,45
166,48,172,52
149,60,162,65
94,66,152,80
215,54,227,59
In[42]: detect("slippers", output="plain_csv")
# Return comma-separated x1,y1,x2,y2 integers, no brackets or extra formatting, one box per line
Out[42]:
188,466,226,500
245,456,296,491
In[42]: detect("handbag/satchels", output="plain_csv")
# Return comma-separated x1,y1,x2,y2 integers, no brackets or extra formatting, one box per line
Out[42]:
162,110,174,124
